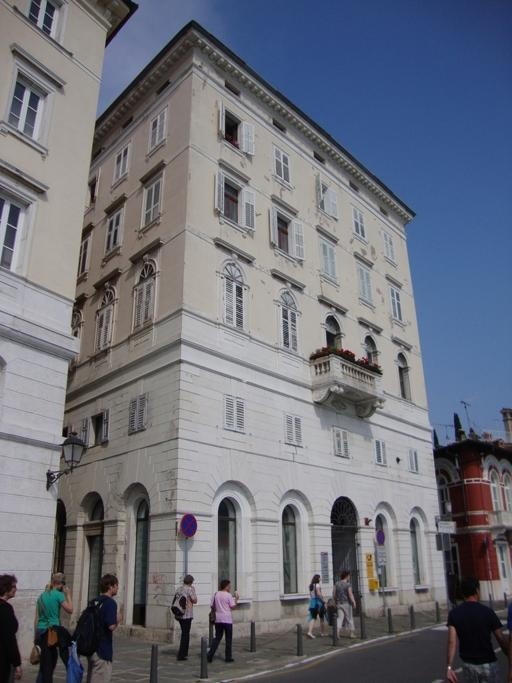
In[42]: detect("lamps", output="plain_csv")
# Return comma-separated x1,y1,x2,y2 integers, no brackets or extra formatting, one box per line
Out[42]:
46,432,88,491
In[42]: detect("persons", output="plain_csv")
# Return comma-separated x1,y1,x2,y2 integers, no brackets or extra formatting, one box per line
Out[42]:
75,573,125,683
206,580,240,662
32,571,73,682
443,576,510,682
170,573,198,661
333,569,358,641
306,573,329,639
0,571,24,683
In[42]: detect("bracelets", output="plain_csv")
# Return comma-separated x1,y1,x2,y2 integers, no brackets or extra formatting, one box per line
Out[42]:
446,664,455,671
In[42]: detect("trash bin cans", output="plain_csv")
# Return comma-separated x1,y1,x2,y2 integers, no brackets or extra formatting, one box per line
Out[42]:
327,598,336,625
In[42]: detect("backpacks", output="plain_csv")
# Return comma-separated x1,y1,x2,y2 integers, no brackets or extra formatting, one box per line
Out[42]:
170,587,192,617
75,596,112,658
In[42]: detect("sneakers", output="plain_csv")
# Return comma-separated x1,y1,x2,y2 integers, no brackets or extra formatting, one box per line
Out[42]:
306,632,316,639
176,657,188,661
224,658,235,663
320,632,329,637
206,652,213,664
349,634,357,638
336,634,341,640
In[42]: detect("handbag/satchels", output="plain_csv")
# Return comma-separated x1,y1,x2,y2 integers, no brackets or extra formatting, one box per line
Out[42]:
47,626,60,647
315,603,321,613
327,605,347,627
29,643,42,665
320,605,327,615
208,605,217,624
65,641,85,683
